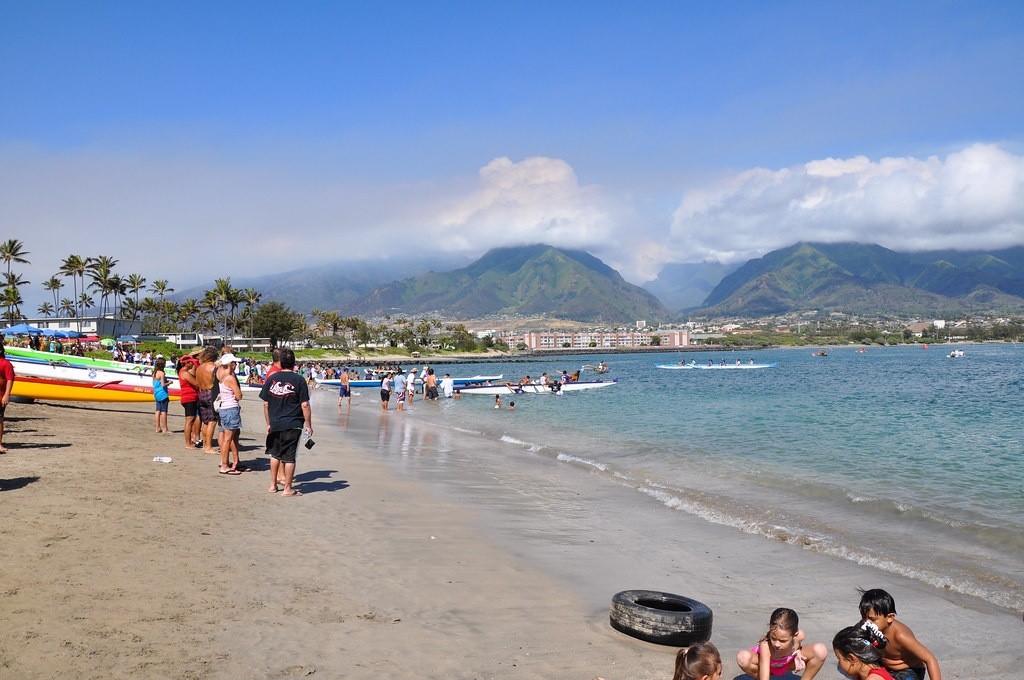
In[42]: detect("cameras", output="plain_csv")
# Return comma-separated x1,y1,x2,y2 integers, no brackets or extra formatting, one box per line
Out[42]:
305,439,315,449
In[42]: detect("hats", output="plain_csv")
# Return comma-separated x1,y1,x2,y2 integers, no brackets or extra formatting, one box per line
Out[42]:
344,367,349,371
411,368,418,372
221,353,242,365
189,346,205,356
180,355,198,363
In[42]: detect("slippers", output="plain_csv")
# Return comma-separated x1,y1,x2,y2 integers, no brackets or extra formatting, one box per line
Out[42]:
283,491,304,497
219,469,242,475
268,486,278,493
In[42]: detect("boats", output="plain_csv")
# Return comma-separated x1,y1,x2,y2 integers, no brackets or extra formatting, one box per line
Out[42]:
655,362,778,370
453,377,618,395
314,373,505,388
3,344,182,404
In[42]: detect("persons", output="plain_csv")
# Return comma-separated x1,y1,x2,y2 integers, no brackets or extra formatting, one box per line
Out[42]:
504,369,580,397
673,640,724,680
236,354,270,381
0,336,15,455
113,342,164,367
708,358,754,366
599,360,606,367
833,619,897,680
678,358,696,365
381,364,460,410
376,362,401,370
338,366,351,407
175,345,251,476
858,588,942,680
736,607,828,680
258,349,313,497
26,331,102,358
465,379,492,387
152,356,175,434
292,360,376,380
495,394,514,409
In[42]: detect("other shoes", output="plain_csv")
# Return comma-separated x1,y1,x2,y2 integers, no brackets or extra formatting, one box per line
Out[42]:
219,463,233,468
277,479,293,486
199,441,203,445
217,448,220,452
195,442,203,449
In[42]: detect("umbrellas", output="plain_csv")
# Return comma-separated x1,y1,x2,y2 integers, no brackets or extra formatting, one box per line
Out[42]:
100,338,116,346
0,323,83,340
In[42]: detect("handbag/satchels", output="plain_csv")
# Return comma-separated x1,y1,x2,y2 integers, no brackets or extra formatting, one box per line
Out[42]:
213,394,222,412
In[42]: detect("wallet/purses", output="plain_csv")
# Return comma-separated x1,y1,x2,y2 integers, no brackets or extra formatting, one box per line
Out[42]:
305,439,315,450
